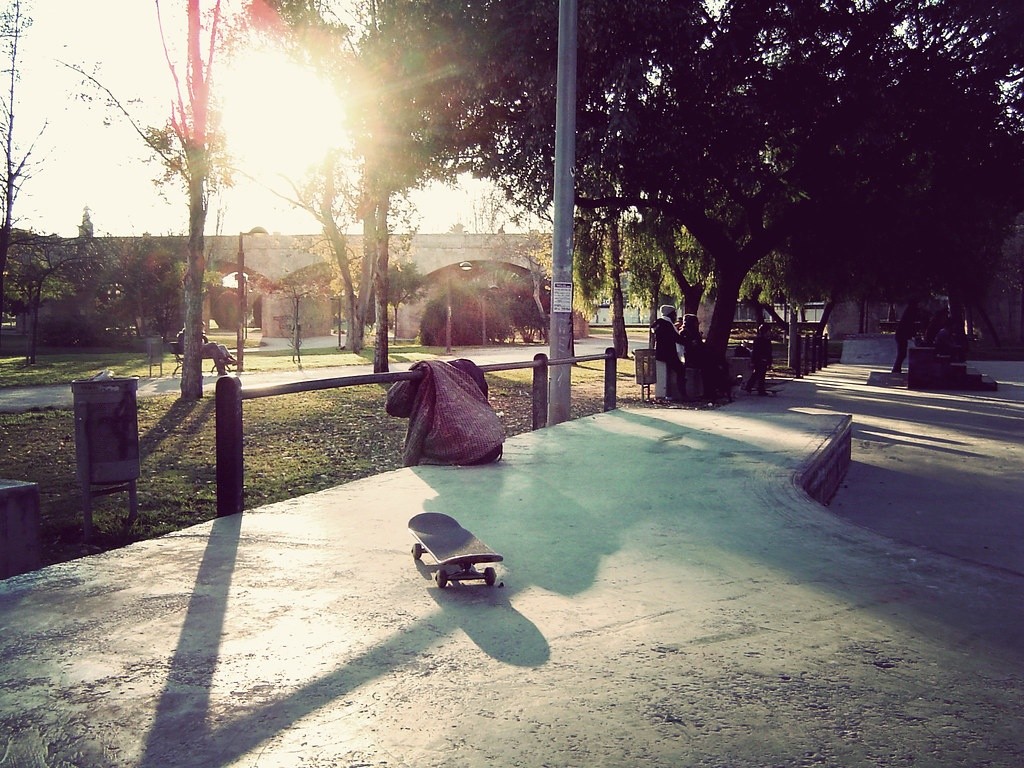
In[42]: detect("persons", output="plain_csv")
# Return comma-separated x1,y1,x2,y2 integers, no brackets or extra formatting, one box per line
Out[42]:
651,305,693,399
178,321,236,375
894,297,967,372
743,324,772,394
676,313,721,400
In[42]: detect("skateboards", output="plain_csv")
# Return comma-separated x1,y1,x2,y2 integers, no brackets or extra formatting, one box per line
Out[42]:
407,512,505,590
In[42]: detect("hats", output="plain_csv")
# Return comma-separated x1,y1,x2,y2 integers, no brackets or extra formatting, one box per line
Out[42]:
661,305,675,316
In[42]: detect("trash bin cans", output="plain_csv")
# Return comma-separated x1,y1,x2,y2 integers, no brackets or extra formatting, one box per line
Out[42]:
634,349,657,384
70,378,142,490
145,337,164,365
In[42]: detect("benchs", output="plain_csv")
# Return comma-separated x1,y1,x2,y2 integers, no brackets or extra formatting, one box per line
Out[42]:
168,342,231,376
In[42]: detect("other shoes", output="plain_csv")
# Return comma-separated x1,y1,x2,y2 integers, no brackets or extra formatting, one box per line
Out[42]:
218,372,228,375
747,384,751,392
759,390,768,395
225,359,234,364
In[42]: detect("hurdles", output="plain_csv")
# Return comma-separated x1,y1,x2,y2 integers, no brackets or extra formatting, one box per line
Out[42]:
740,386,783,397
